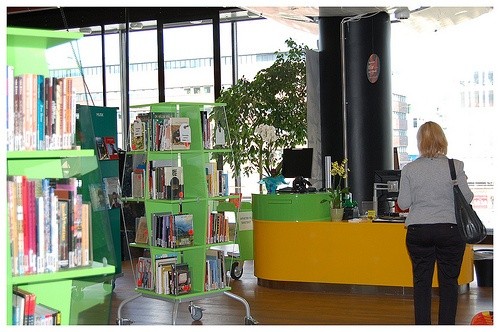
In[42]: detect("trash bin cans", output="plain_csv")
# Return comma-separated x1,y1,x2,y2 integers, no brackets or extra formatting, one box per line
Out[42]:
473,248,493,288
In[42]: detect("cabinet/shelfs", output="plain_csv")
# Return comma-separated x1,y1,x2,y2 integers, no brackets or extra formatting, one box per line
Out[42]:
116,102,258,325
76,104,124,282
7,27,115,326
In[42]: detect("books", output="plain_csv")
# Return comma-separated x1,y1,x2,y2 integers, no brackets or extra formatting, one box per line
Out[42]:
6,66,77,152
89,161,229,213
137,253,231,297
8,175,90,277
134,211,231,249
13,289,61,326
95,111,213,160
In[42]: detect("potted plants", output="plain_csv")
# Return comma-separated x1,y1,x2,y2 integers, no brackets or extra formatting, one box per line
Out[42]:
343,198,358,219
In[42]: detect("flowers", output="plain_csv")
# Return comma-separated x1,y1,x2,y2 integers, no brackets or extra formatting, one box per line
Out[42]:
320,158,351,209
255,123,279,178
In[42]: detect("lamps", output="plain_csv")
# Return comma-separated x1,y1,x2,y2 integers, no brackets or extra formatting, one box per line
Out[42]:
130,23,143,30
395,8,410,19
79,28,92,34
191,20,202,24
247,11,260,18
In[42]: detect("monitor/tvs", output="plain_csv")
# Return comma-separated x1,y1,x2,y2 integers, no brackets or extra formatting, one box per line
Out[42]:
373,170,401,197
282,148,313,178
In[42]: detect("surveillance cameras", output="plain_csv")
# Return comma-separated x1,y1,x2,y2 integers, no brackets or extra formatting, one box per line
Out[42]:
394,7,409,19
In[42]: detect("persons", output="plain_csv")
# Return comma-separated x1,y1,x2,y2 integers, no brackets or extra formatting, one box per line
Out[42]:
397,122,474,325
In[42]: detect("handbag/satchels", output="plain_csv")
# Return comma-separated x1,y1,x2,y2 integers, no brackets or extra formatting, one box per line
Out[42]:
448,159,487,244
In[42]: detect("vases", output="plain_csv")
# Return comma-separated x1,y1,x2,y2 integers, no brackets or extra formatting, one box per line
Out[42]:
330,209,344,222
257,176,290,194
